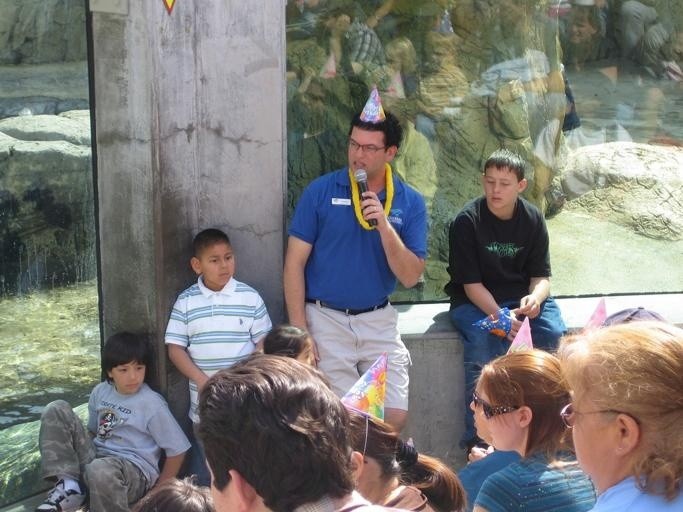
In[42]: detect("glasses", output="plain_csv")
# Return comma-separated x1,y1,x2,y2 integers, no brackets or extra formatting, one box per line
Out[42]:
472,393,519,419
349,139,385,152
559,402,640,428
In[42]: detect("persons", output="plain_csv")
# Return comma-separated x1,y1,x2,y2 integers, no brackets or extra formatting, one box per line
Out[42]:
446,148,568,452
36,331,192,512
164,229,272,487
456,308,683,510
127,305,467,512
282,83,428,432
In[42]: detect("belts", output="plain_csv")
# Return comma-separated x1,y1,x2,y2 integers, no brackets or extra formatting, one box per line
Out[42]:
304,298,388,315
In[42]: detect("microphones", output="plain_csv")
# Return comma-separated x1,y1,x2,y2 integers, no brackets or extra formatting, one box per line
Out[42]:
352,168,378,227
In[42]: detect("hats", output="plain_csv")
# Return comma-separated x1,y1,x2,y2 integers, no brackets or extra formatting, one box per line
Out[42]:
473,307,512,337
601,306,667,327
506,316,533,355
339,352,387,423
585,298,607,331
360,85,387,124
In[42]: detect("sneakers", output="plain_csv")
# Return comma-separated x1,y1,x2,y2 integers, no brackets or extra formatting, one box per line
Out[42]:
34,478,82,512
